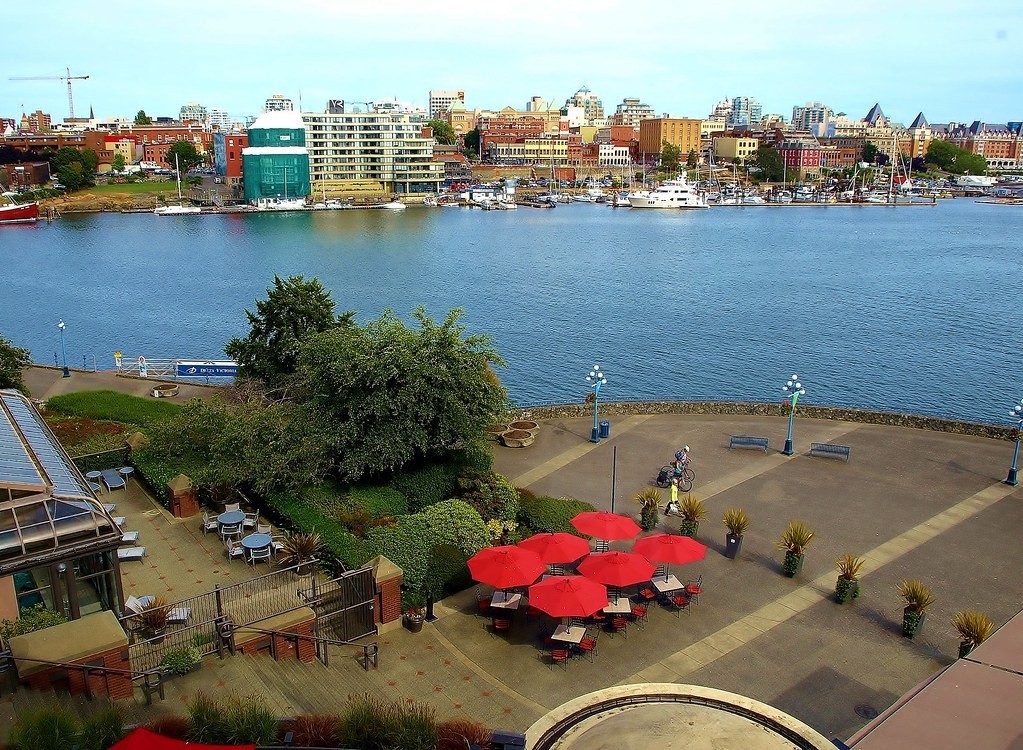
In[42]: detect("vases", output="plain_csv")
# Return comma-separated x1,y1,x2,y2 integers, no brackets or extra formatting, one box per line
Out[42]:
406,618,422,633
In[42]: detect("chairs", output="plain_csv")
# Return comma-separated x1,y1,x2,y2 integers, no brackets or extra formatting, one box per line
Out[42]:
87,481,102,496
101,469,126,494
124,595,192,628
102,504,146,565
201,503,289,572
475,540,703,671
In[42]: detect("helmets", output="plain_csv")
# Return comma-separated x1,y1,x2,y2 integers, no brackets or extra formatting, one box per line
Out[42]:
684,445,689,452
678,456,685,460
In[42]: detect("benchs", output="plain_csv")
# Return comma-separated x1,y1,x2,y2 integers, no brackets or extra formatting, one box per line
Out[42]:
811,443,850,464
729,434,769,455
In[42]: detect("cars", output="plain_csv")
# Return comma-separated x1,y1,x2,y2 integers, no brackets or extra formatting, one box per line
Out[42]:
92,169,214,176
480,172,646,188
825,165,952,188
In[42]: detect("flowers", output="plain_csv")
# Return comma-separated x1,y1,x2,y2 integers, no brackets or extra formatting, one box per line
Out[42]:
585,392,595,405
778,401,793,417
1006,427,1021,443
405,607,426,621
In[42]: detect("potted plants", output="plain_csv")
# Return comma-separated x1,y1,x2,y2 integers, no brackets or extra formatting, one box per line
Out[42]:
128,595,176,645
834,552,866,605
275,526,325,576
636,488,662,532
158,646,203,682
678,492,710,539
772,520,816,578
722,507,749,559
948,609,994,659
895,578,936,640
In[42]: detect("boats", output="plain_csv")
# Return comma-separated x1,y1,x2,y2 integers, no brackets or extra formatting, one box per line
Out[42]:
498,197,518,209
584,178,956,209
560,193,572,203
574,195,596,201
539,195,557,203
423,196,438,207
438,203,460,207
0,184,38,224
481,200,495,210
383,200,407,209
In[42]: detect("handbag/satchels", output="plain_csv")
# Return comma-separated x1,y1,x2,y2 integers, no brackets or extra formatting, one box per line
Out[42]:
669,503,678,514
658,471,668,482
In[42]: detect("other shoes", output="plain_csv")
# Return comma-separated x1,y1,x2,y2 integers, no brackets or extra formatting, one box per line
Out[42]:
664,513,670,517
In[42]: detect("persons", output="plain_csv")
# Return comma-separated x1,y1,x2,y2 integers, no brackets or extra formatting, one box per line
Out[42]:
664,446,691,517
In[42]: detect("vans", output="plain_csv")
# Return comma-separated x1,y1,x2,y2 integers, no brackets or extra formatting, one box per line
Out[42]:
54,184,67,190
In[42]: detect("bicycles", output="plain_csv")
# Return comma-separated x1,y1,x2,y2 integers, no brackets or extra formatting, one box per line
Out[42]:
661,457,696,482
656,469,693,492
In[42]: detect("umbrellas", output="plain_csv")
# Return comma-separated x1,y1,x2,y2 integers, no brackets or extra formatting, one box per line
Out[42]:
528,574,609,634
569,511,642,552
576,549,658,605
467,545,547,600
631,534,708,583
517,530,590,576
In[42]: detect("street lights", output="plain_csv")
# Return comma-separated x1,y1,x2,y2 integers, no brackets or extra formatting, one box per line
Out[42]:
1005,399,1023,485
781,374,806,455
58,318,71,377
585,364,607,443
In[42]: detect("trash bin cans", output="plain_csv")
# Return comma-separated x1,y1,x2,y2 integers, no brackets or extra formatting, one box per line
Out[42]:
599,419,610,438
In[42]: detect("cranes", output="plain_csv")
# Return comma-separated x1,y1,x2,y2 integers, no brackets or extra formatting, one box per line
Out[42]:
8,67,90,134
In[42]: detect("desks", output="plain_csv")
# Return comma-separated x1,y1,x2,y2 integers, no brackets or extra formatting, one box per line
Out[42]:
242,533,272,565
217,511,246,541
119,467,134,482
86,471,101,484
550,624,587,661
490,590,522,625
603,598,632,633
651,574,685,606
